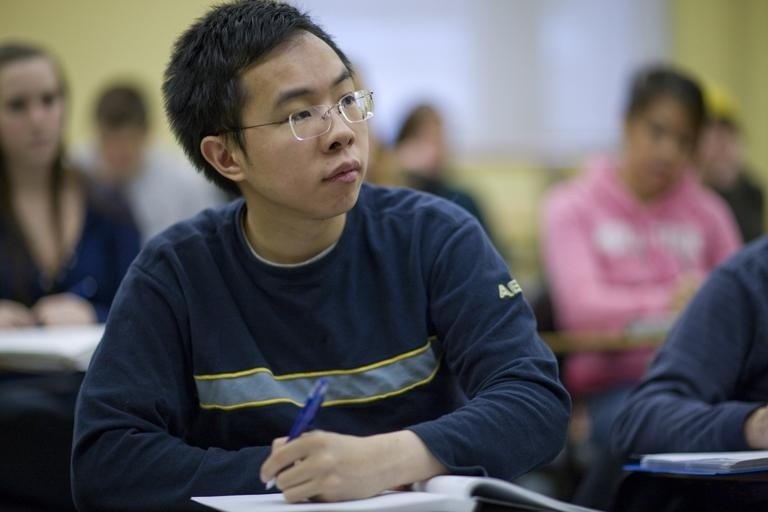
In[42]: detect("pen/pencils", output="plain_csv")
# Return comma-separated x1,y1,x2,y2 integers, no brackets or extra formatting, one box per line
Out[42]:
265,377,330,491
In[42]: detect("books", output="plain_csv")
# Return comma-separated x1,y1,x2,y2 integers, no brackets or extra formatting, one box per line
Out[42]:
190,475,600,511
637,450,766,472
0,326,108,377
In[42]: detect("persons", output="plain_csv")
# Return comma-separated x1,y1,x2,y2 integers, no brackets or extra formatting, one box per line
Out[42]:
397,102,492,255
698,105,763,249
538,63,745,454
1,44,141,511
605,232,767,467
70,0,571,511
67,83,223,249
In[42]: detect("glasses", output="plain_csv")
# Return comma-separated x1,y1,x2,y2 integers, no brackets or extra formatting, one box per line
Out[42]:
236,90,377,142
